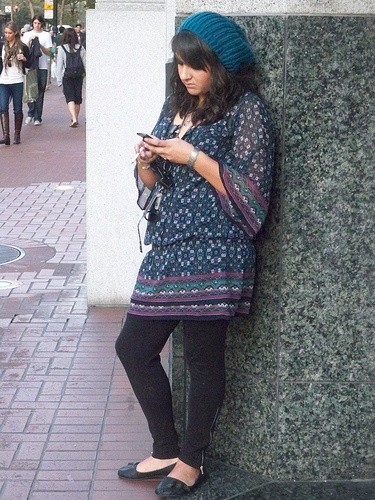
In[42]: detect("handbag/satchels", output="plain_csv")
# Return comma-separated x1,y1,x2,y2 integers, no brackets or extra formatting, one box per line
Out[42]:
23,70,40,103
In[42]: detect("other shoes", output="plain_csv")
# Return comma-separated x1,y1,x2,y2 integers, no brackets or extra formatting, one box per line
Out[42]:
69,119,78,126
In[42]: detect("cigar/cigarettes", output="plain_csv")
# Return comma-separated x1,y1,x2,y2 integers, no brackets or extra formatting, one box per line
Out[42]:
131,147,144,164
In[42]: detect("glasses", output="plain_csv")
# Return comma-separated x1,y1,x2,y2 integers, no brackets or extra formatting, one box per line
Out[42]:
8,59,12,67
137,170,174,253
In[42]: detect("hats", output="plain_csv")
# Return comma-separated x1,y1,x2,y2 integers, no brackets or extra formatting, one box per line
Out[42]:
177,10,256,72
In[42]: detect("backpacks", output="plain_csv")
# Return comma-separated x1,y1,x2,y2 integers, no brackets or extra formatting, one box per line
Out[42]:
60,43,86,79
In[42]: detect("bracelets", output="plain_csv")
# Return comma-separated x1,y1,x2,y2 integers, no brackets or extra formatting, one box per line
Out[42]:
187,147,201,169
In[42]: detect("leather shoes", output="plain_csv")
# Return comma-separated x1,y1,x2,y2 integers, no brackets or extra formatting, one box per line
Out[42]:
155,468,210,496
118,459,178,481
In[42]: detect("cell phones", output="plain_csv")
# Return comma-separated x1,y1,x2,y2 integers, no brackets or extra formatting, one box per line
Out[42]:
137,132,153,139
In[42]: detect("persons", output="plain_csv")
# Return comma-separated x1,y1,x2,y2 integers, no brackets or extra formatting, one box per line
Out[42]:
114,10,275,497
19,16,86,126
0,22,32,146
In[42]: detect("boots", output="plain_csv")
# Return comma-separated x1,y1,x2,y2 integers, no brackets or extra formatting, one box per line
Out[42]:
13,113,23,144
0,112,11,145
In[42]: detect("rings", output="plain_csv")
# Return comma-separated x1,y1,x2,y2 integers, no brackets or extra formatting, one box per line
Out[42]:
163,158,167,161
